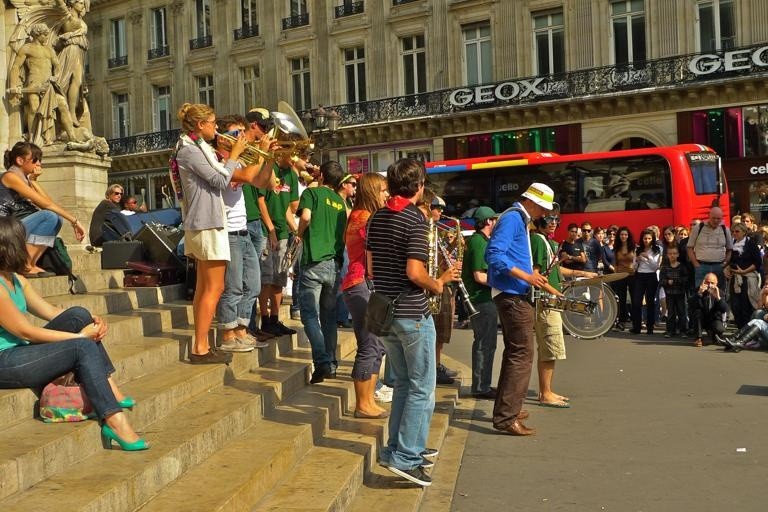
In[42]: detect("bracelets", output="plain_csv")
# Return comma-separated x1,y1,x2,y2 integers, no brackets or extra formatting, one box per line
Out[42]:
72,219,78,228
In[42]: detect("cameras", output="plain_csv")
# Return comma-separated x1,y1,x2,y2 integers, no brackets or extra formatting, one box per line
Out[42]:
708,282,714,289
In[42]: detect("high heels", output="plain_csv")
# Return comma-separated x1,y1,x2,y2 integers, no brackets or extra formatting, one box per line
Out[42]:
100,423,152,451
117,393,139,410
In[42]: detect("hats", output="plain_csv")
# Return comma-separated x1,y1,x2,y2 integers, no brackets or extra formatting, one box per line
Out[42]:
471,205,500,220
520,182,555,212
431,194,447,207
245,107,276,127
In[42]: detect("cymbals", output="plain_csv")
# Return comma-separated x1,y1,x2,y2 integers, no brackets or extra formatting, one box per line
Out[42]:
574,272,630,285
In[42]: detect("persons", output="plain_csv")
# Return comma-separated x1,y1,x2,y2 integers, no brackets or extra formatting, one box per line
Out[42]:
54,127,109,155
0,141,85,278
0,218,152,450
11,23,81,144
88,184,130,246
119,195,148,216
57,0,90,126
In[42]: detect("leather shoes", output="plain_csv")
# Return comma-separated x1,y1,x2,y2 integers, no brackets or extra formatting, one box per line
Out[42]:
494,419,538,435
190,347,232,364
46,271,56,277
21,271,50,279
513,408,531,419
353,407,391,419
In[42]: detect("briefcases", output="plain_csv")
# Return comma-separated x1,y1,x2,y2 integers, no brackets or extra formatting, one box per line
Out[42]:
100,232,146,270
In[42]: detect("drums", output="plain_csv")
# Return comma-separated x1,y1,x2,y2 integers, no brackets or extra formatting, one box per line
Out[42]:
542,278,620,338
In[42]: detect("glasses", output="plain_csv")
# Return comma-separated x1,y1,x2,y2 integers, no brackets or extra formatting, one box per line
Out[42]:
544,214,562,225
30,157,41,164
343,182,357,188
111,191,123,196
581,228,591,233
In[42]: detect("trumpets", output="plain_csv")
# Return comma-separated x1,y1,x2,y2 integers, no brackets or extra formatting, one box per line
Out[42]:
214,131,275,165
254,137,315,161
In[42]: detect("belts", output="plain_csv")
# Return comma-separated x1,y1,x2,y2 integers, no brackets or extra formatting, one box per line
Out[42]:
697,260,723,266
226,229,250,236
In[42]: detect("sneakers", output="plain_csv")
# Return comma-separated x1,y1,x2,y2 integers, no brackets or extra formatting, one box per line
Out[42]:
308,362,330,386
253,326,276,340
373,389,393,404
695,338,703,348
219,336,255,352
436,363,458,384
240,333,268,348
261,320,287,337
419,447,439,457
328,360,339,380
418,454,434,469
472,387,499,399
248,328,269,342
271,319,297,335
387,461,433,487
379,384,394,392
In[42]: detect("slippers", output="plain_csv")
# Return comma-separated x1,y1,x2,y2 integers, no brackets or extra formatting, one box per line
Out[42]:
539,400,570,409
538,393,571,403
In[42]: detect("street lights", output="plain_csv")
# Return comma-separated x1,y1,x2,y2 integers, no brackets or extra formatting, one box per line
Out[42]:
300,102,342,175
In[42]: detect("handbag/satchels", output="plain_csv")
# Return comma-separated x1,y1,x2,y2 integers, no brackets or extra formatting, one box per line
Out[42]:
38,235,73,276
38,369,98,423
0,194,39,221
363,291,399,338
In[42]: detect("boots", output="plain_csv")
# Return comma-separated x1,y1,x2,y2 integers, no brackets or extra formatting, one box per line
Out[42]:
713,324,752,349
726,323,760,353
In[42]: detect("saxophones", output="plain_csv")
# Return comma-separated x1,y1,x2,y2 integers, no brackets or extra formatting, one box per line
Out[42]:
440,215,464,274
422,202,442,314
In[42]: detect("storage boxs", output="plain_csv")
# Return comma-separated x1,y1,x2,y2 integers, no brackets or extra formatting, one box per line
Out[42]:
122,260,159,287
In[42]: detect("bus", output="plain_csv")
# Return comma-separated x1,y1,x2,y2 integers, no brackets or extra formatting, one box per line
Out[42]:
422,140,734,244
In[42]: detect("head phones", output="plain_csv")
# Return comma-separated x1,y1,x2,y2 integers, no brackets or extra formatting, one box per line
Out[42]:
540,202,560,231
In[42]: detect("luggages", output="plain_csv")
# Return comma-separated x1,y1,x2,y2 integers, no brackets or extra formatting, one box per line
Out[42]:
122,259,183,288
